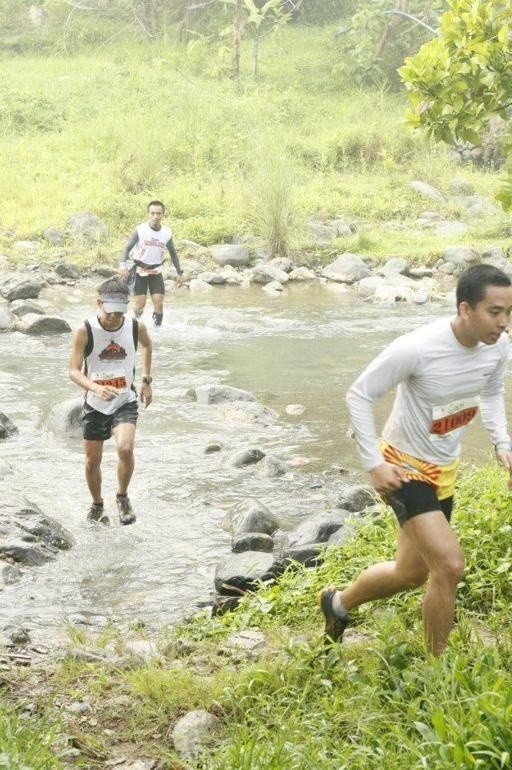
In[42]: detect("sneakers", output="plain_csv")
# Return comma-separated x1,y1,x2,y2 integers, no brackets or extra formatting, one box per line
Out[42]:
87,504,103,521
316,586,352,657
116,497,135,523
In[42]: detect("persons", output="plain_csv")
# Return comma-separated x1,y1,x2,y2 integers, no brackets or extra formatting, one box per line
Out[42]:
319,264,511,655
120,199,185,326
68,275,152,527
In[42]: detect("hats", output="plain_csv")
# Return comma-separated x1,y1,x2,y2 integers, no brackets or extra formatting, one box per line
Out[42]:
98,292,128,314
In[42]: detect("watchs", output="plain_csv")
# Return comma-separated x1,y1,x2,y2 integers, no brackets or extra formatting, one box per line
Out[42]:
142,376,152,384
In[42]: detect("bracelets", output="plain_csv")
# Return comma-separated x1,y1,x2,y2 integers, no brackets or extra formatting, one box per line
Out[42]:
177,270,184,274
